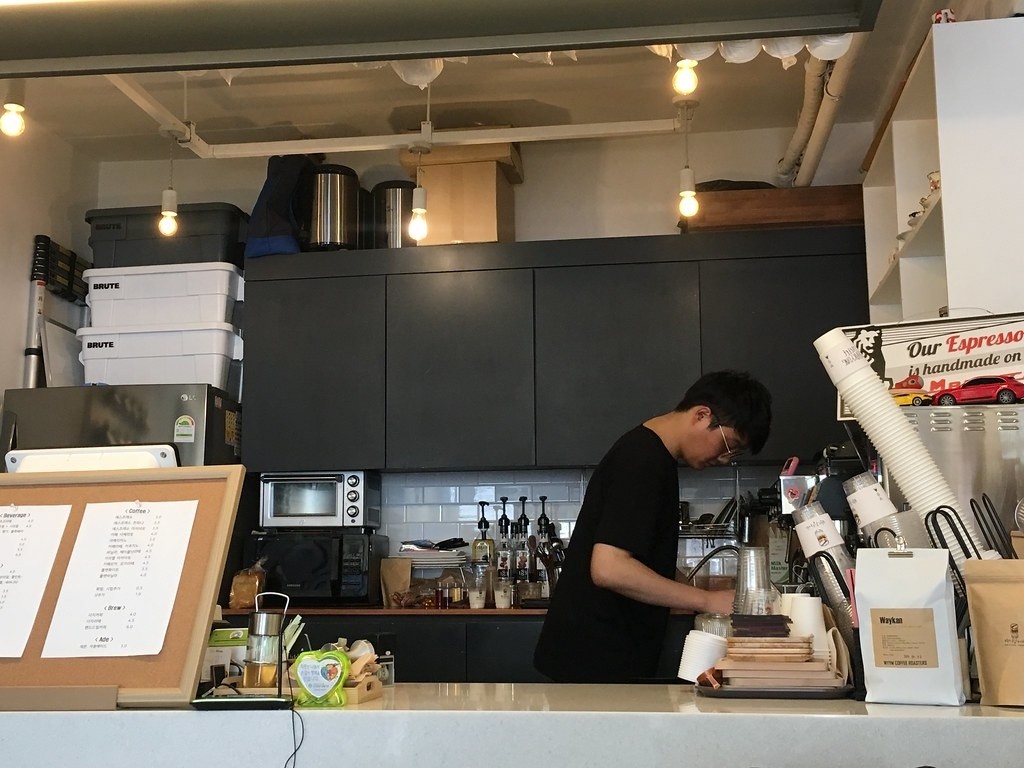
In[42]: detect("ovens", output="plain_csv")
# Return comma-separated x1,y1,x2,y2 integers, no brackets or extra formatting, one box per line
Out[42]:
258,470,380,532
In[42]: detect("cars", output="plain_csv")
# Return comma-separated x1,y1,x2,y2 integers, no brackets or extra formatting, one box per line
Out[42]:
891,391,931,406
933,372,1024,407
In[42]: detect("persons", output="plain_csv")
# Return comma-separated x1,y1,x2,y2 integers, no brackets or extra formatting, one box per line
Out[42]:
534,370,773,684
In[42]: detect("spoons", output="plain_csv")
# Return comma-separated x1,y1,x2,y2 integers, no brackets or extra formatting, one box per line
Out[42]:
695,666,720,690
546,538,563,557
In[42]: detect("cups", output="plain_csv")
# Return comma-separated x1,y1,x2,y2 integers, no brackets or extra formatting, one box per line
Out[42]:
518,582,539,604
927,170,941,192
841,472,931,555
418,584,434,608
782,594,832,669
790,500,856,652
466,577,486,608
493,576,512,609
732,547,780,614
692,611,730,639
814,328,990,601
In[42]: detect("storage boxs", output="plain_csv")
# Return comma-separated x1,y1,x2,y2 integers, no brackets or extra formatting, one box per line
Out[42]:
685,184,865,231
400,120,527,247
71,202,250,402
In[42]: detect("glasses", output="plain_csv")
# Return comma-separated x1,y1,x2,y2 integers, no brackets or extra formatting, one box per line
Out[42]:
711,412,744,466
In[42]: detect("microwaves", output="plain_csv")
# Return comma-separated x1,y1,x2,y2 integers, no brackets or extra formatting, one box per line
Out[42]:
256,534,389,607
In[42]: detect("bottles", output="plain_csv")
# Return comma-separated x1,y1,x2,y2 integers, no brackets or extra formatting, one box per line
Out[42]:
243,612,281,687
470,495,554,606
436,581,464,608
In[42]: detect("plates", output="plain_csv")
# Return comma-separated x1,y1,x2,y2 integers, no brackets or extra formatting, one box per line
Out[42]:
728,636,815,663
896,193,938,240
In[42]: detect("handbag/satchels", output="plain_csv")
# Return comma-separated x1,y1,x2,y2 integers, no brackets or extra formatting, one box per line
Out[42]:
855,548,966,705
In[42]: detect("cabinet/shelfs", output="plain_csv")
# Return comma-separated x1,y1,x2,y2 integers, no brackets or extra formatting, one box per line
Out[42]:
862,16,1024,326
240,225,885,475
226,613,700,684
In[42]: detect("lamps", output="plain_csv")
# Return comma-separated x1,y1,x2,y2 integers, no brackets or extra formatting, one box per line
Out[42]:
158,123,185,238
672,93,701,218
672,48,700,96
0,78,26,137
408,142,432,243
